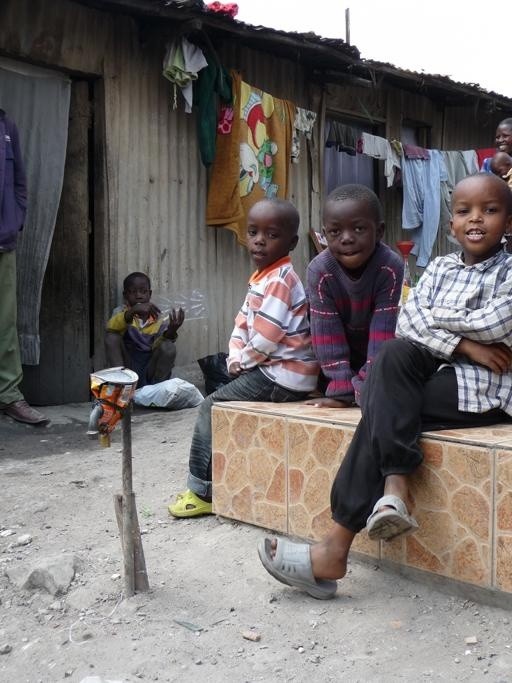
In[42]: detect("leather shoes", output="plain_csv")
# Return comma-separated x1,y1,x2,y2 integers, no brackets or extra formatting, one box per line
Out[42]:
1,401,48,423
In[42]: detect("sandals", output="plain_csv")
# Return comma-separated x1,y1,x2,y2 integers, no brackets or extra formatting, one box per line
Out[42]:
168,490,213,517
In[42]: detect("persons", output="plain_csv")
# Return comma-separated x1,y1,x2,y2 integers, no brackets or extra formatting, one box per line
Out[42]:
104,271,185,390
167,196,320,518
252,171,511,601
491,151,512,187
478,117,512,175
0,103,52,427
304,182,406,411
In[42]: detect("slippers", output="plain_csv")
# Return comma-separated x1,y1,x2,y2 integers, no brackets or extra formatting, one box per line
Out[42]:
366,495,419,541
257,538,336,599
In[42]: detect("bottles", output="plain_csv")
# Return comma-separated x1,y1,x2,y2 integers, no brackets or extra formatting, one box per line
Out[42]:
403,257,410,278
